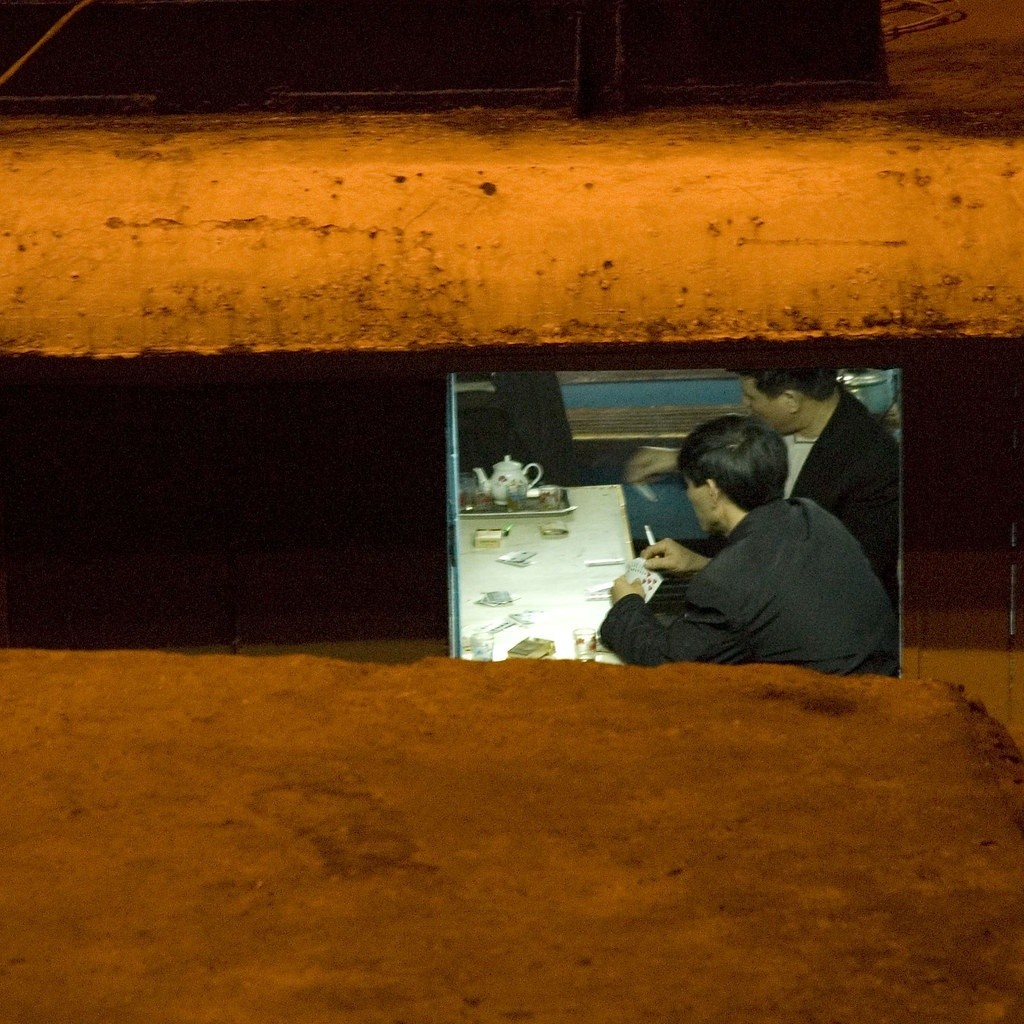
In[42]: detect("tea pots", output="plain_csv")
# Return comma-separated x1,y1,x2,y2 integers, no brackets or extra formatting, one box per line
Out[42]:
472,454,544,506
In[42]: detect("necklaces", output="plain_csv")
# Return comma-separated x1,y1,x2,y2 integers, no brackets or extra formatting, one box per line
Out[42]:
793,434,816,444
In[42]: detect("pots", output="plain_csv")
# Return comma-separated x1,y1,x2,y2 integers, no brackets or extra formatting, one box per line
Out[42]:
837,368,900,415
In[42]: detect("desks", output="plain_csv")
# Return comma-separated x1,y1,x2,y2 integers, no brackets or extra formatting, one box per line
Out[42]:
459,484,640,664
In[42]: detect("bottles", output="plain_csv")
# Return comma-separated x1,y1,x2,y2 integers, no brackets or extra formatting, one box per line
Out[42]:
471,632,495,661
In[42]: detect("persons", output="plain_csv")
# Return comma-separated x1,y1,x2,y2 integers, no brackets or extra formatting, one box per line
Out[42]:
600,414,896,674
618,367,898,597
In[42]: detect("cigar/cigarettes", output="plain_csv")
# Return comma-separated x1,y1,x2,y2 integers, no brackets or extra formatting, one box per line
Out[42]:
644,525,659,558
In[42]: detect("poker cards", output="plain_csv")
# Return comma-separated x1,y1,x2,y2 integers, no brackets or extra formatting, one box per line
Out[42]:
473,589,520,608
499,548,540,568
510,607,545,625
622,556,665,605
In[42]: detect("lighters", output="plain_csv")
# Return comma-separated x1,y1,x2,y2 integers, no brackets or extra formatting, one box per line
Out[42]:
500,521,513,534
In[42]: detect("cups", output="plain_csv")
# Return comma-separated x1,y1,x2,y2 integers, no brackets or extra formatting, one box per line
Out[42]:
573,627,597,662
472,486,492,511
538,485,560,510
507,484,526,511
